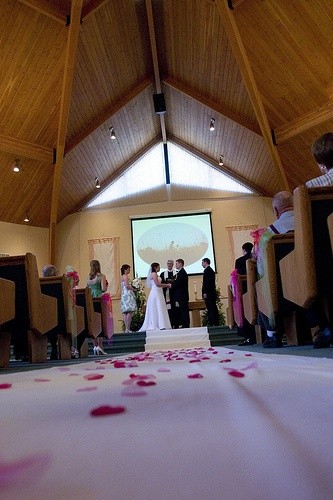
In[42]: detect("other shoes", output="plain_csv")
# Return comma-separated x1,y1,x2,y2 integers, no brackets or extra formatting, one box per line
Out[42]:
72,348,80,359
238,337,255,345
125,329,135,333
262,332,284,348
311,327,332,349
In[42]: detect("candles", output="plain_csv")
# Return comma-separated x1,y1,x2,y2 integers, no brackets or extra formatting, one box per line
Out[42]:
194,281,196,293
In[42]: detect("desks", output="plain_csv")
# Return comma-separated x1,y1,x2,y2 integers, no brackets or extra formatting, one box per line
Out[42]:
143,301,207,327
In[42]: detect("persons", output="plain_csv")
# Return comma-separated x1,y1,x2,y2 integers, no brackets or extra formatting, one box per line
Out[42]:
306,132,333,349
136,262,172,332
257,191,295,348
160,260,179,329
167,259,190,328
202,258,220,327
86,260,107,355
10,265,87,363
121,264,140,333
235,242,257,346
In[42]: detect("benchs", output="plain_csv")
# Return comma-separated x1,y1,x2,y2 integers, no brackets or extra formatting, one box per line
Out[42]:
226,186,333,346
0,253,114,368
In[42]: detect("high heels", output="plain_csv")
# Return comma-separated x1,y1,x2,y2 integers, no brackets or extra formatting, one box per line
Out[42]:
93,346,107,355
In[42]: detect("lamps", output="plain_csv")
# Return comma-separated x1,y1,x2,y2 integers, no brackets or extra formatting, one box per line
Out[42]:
109,127,115,140
95,177,100,189
219,155,223,165
24,211,29,221
210,118,215,131
14,159,19,173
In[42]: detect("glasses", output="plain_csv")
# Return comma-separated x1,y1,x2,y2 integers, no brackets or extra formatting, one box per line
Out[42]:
168,263,172,264
